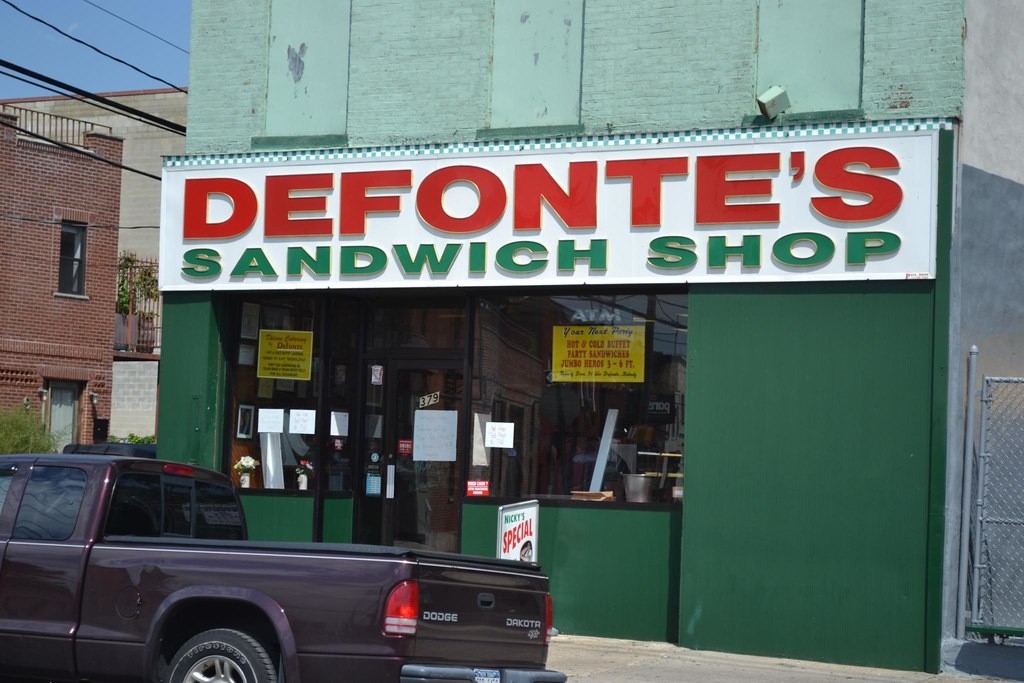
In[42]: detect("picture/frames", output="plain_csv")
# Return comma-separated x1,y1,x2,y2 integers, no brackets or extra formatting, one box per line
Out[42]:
236,401,256,440
301,315,316,336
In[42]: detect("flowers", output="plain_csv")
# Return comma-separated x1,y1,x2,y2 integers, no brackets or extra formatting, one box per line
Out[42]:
296,460,317,482
234,456,260,483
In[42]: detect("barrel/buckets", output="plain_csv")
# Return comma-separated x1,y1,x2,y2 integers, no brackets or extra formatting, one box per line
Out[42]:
622,472,658,503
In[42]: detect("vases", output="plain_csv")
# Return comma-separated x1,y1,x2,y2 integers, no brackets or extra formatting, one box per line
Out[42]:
299,474,307,490
241,473,250,488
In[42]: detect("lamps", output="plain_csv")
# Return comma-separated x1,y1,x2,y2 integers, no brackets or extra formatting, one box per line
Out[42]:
89,391,98,405
38,389,48,401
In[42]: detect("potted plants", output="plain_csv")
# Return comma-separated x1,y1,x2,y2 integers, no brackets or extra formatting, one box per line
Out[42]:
113,250,163,353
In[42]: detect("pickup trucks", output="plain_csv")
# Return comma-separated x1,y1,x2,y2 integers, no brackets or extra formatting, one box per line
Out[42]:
0,454,569,683
